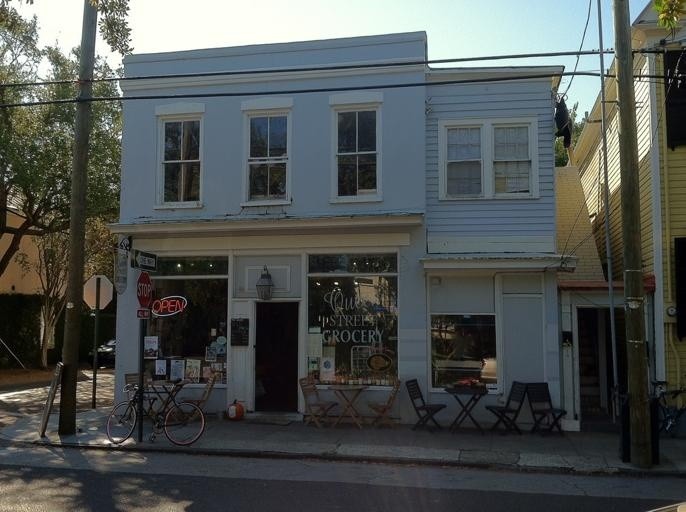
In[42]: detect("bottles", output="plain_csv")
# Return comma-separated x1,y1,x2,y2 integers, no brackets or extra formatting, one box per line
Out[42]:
312,372,397,386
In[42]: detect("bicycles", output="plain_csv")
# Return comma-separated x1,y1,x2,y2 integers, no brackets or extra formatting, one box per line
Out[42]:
647,375,686,440
105,378,206,446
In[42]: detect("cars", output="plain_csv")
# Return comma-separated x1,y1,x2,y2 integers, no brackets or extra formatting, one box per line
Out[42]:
476,354,497,384
92,341,114,369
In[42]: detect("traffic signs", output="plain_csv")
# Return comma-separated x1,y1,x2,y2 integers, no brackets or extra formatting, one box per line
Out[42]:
131,248,157,272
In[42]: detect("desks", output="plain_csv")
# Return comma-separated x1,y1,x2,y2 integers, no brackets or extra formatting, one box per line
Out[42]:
445,387,488,434
148,378,187,420
325,383,369,430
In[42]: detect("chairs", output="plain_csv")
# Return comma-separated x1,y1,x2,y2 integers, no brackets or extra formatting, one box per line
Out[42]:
404,378,445,436
177,376,216,421
484,380,527,437
367,381,400,428
524,382,568,441
297,375,337,431
124,373,155,419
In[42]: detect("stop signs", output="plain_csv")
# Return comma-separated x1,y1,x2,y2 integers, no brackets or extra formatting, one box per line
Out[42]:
133,271,153,310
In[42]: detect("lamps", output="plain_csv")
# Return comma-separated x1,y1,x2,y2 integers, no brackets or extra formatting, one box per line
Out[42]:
255,264,274,298
